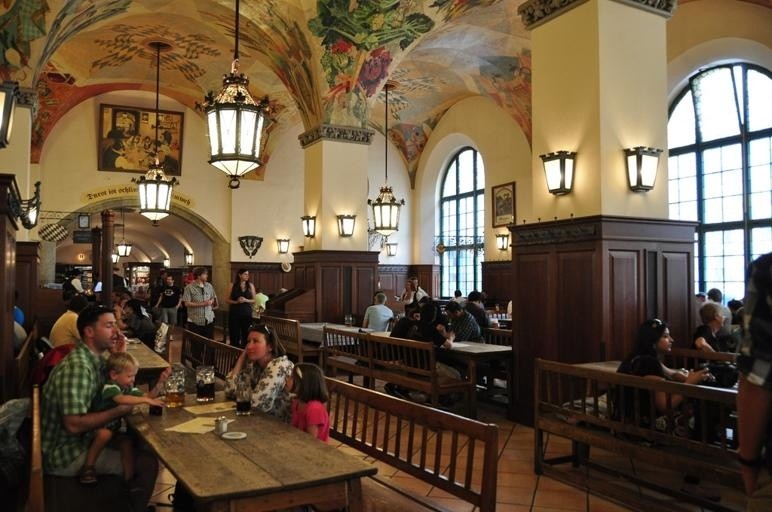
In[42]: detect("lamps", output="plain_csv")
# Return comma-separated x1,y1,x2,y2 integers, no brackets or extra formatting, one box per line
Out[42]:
277,238,289,255
301,217,315,239
78,249,84,262
385,242,398,256
336,213,357,237
496,233,510,251
115,207,137,258
199,0,277,189
626,146,664,194
136,42,179,227
163,258,169,267
366,80,405,248
111,253,120,263
539,150,579,196
184,254,193,264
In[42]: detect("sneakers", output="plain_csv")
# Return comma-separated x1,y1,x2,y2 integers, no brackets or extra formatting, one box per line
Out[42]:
384,383,397,396
393,386,411,400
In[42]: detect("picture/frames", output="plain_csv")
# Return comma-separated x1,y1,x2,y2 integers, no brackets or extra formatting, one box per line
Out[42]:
97,102,187,179
492,181,517,228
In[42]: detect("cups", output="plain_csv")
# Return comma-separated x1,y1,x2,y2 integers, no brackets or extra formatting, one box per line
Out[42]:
236,382,251,415
165,370,185,407
344,313,353,327
196,366,216,402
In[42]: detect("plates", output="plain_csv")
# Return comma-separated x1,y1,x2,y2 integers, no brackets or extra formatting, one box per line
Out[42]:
222,431,248,440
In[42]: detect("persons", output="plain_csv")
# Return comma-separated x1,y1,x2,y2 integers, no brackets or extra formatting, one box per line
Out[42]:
450,290,468,308
408,305,461,380
482,289,488,306
39,306,158,512
12,289,29,325
49,294,89,347
103,129,179,170
361,293,394,332
286,363,330,443
119,296,153,327
612,319,715,449
696,288,733,326
80,351,172,493
153,275,182,340
729,299,743,322
383,303,421,399
734,253,772,511
111,267,127,292
465,292,489,327
732,307,746,358
696,292,705,302
692,303,739,364
117,300,160,346
436,301,484,343
400,281,422,316
250,288,270,320
403,277,429,296
63,269,84,305
110,290,122,308
13,320,27,349
364,291,384,313
183,267,219,368
224,268,256,349
121,292,133,307
224,324,295,423
136,286,147,300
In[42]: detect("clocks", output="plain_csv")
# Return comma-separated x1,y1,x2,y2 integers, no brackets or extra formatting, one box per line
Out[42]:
79,215,90,229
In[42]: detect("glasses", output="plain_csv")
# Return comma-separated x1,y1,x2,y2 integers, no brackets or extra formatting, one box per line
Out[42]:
247,322,272,336
646,318,664,332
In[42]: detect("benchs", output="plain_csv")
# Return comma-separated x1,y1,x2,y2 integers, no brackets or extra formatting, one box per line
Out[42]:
16,332,33,397
532,356,770,511
321,374,500,511
30,384,135,512
33,318,42,349
479,327,513,406
261,315,321,363
351,313,399,333
324,326,472,418
664,347,740,378
169,326,245,384
